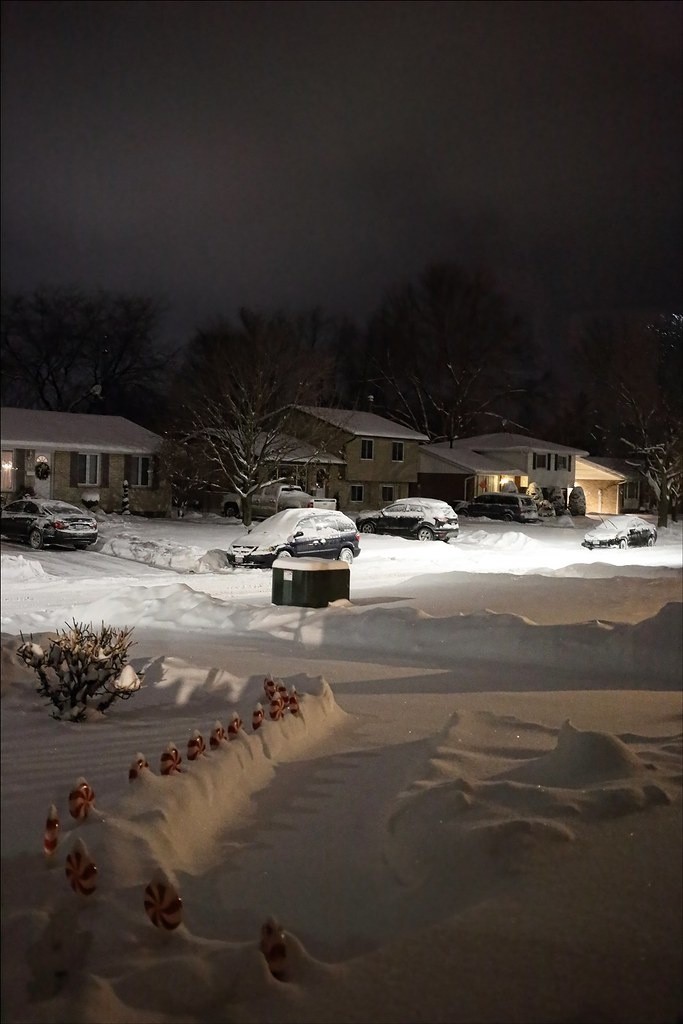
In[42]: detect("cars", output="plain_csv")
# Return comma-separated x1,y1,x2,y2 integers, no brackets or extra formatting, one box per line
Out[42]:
226,507,360,570
355,496,460,541
584,514,658,550
0,498,99,551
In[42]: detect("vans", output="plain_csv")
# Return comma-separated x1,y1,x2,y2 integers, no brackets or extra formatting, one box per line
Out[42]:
455,492,538,523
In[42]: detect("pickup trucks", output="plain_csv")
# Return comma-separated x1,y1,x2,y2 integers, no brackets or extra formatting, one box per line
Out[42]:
220,483,337,518
